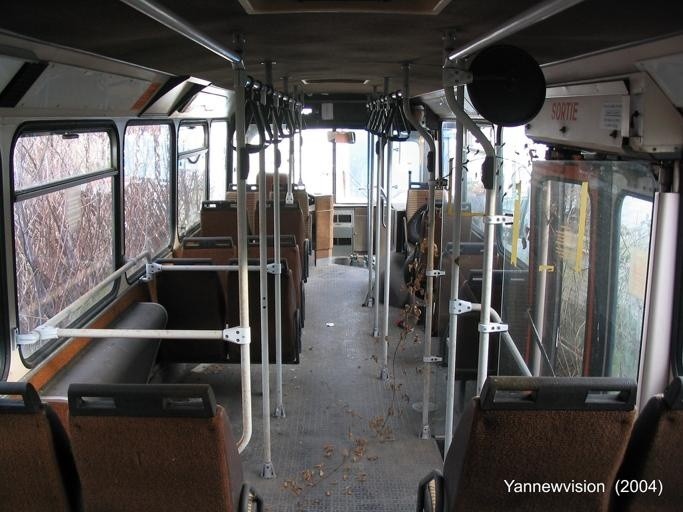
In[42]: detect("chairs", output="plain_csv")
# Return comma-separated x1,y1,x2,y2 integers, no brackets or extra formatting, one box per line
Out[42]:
443,269,530,392
151,258,228,364
256,172,288,200
0,381,80,512
66,382,263,511
254,200,310,283
432,241,501,337
270,184,313,255
416,375,641,512
418,202,471,253
180,236,236,266
224,258,302,364
200,200,253,243
406,182,448,222
615,378,683,512
225,183,259,230
246,234,305,327
